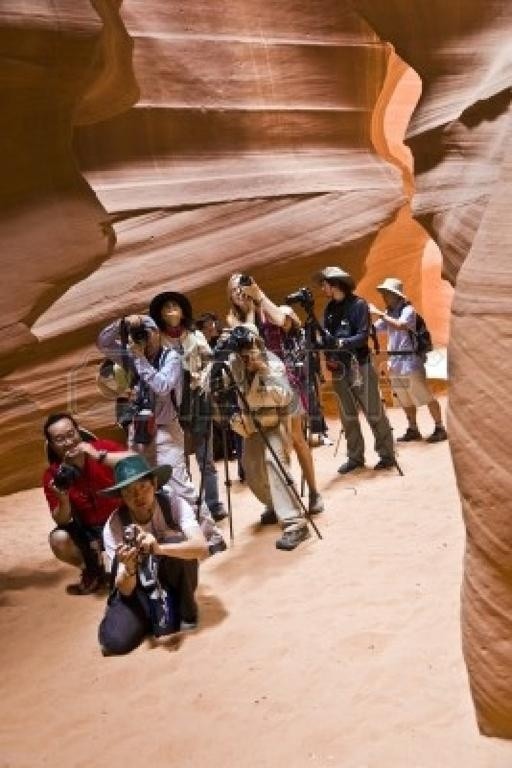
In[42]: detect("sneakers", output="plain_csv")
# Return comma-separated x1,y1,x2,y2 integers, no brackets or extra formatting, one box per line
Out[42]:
427,428,446,441
261,509,277,523
275,526,309,551
66,569,104,594
374,457,396,470
308,492,323,514
397,429,423,442
337,460,364,475
210,504,229,521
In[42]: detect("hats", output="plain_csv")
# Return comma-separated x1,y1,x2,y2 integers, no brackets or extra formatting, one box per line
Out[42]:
195,313,218,328
98,456,172,499
376,278,408,301
279,305,301,328
313,267,356,293
44,428,98,463
148,291,191,331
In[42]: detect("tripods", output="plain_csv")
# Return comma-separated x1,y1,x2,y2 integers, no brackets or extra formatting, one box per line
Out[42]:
300,316,404,497
197,363,324,541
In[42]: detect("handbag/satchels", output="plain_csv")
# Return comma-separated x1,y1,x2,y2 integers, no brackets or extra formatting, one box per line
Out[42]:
229,406,279,439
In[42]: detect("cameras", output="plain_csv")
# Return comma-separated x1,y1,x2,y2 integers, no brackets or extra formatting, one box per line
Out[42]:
54,463,81,490
122,525,136,547
226,326,253,352
240,274,251,286
130,324,148,344
286,287,314,309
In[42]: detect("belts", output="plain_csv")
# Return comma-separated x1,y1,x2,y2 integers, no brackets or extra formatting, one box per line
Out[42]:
386,351,417,356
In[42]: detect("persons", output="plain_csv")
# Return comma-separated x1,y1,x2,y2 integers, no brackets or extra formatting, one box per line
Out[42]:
311,266,396,473
367,277,448,444
98,454,209,653
40,410,137,595
98,272,325,552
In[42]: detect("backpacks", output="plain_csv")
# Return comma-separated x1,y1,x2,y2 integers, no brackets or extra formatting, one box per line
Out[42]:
399,304,434,353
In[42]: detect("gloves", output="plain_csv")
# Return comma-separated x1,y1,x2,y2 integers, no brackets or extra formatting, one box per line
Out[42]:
100,358,116,378
